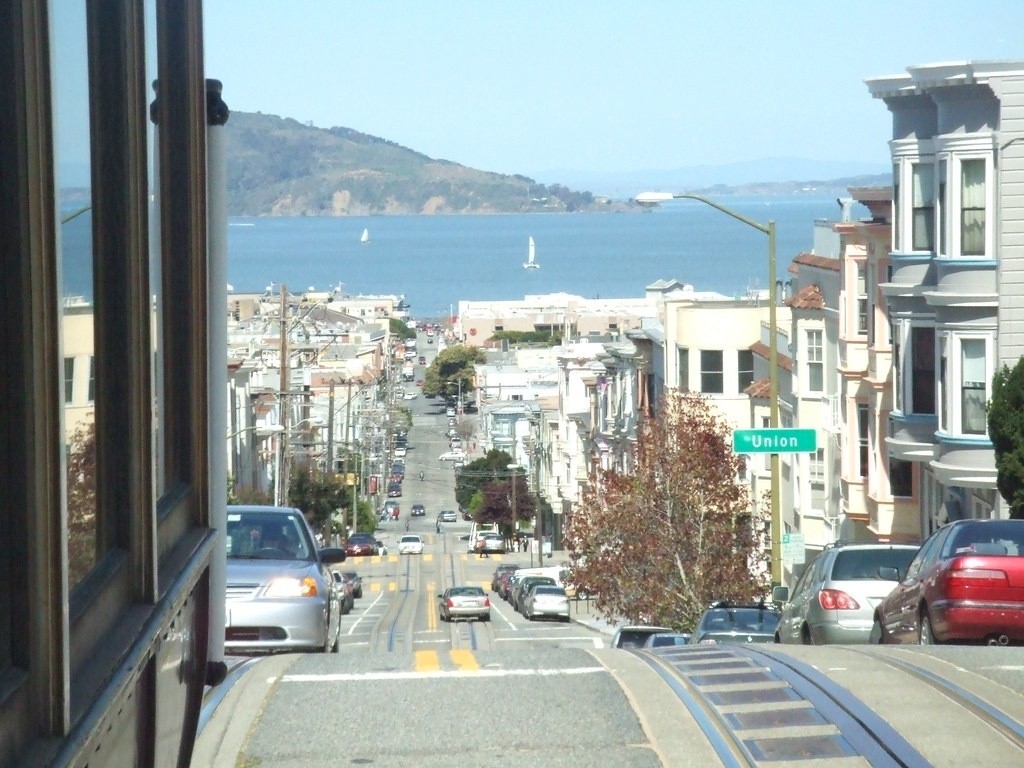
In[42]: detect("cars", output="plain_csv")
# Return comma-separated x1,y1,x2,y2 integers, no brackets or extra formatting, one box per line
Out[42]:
492,563,600,612
397,535,424,554
332,570,362,615
687,604,785,645
403,393,418,400
769,540,925,643
872,519,1024,647
437,586,491,621
437,510,457,522
483,534,506,554
445,406,463,452
222,503,347,656
386,458,405,496
396,392,403,398
351,533,389,555
611,624,677,650
640,633,694,649
396,440,409,449
382,501,400,522
402,324,436,398
438,451,468,463
522,585,571,623
462,511,474,521
474,531,494,549
346,537,374,556
411,505,425,516
394,447,407,457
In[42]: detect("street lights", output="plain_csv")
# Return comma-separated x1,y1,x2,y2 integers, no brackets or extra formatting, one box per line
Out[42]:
633,191,783,606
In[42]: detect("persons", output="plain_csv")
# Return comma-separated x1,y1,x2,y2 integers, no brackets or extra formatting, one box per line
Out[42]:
479,536,488,558
516,534,529,552
436,516,441,533
473,441,476,449
255,521,296,559
405,520,410,532
420,471,424,480
395,508,399,521
